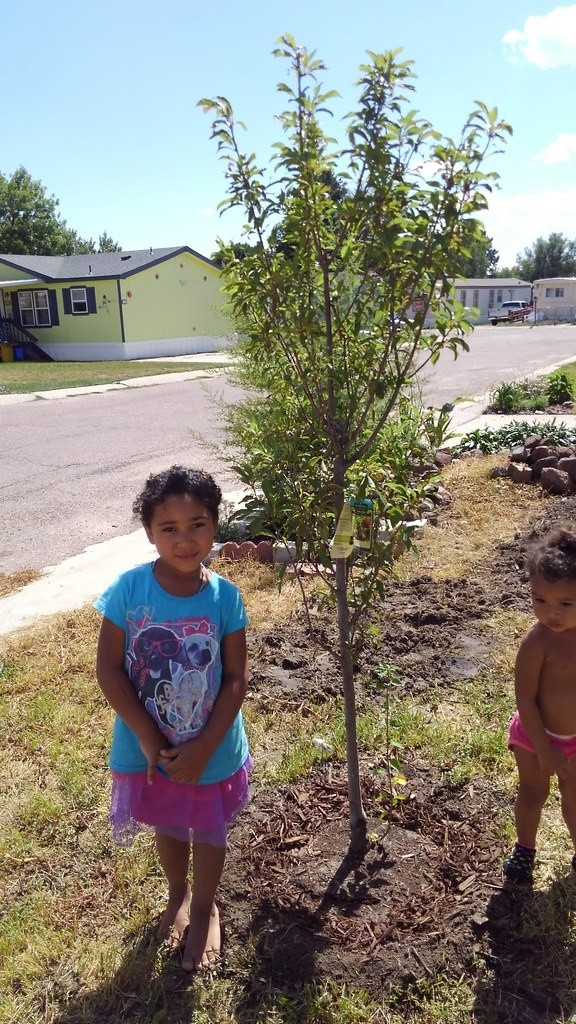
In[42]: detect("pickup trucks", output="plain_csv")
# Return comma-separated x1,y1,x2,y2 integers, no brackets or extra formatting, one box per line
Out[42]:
488,300,529,326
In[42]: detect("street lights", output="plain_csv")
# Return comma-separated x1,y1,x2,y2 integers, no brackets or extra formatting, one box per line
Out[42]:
534,295,538,322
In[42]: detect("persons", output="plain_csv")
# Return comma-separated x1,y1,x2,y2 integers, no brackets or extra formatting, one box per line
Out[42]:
502,519,576,878
93,464,254,971
